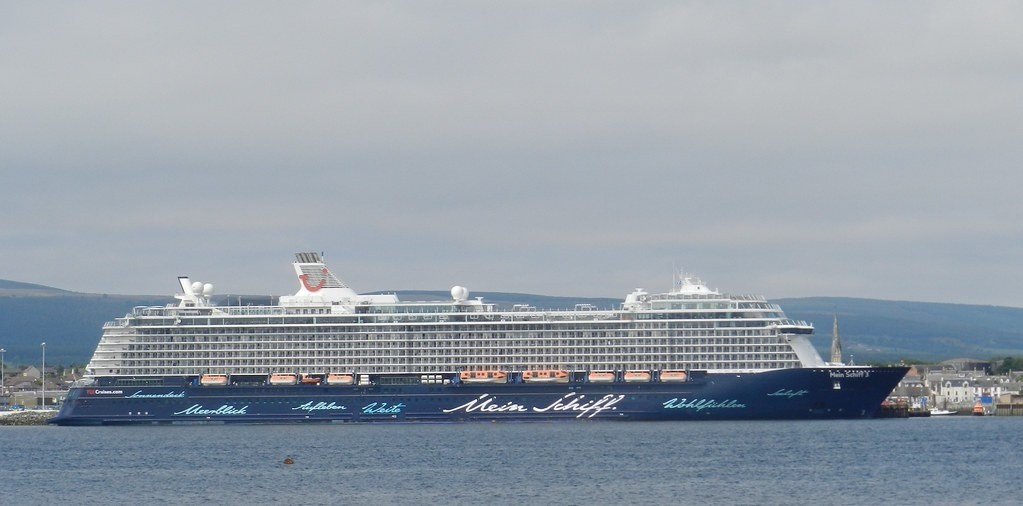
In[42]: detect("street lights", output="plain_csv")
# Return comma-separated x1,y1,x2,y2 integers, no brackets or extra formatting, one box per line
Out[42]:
41,343,45,410
0,349,7,398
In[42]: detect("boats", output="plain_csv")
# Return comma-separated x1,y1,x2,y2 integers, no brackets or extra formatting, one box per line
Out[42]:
270,372,297,385
201,374,227,385
521,370,570,383
589,372,615,383
326,373,354,385
459,370,508,384
300,373,322,384
928,407,958,415
623,370,651,383
658,371,687,383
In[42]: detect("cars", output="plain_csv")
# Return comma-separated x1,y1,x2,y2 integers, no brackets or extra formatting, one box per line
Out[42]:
10,404,25,412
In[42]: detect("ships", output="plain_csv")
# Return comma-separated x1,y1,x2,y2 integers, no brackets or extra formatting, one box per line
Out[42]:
42,253,913,428
